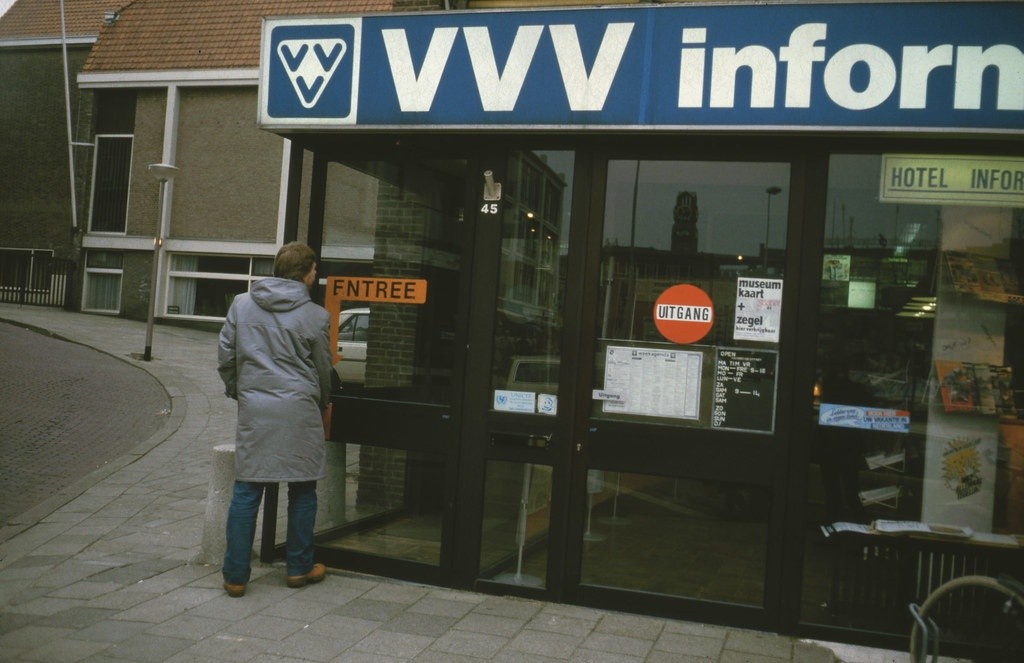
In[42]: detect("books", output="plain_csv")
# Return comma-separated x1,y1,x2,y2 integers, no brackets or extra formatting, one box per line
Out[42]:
935,358,1019,422
944,249,1021,294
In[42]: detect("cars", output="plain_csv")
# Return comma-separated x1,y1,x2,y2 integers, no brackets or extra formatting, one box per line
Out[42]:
336,306,370,361
504,354,560,395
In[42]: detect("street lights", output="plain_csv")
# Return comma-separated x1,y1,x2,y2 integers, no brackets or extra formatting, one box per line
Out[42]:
764,184,784,277
143,162,180,361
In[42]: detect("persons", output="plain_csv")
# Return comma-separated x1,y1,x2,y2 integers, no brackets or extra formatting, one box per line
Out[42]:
216,241,332,597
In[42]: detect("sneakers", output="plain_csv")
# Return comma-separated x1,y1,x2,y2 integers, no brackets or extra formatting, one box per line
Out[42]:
223,581,245,597
287,563,325,588
